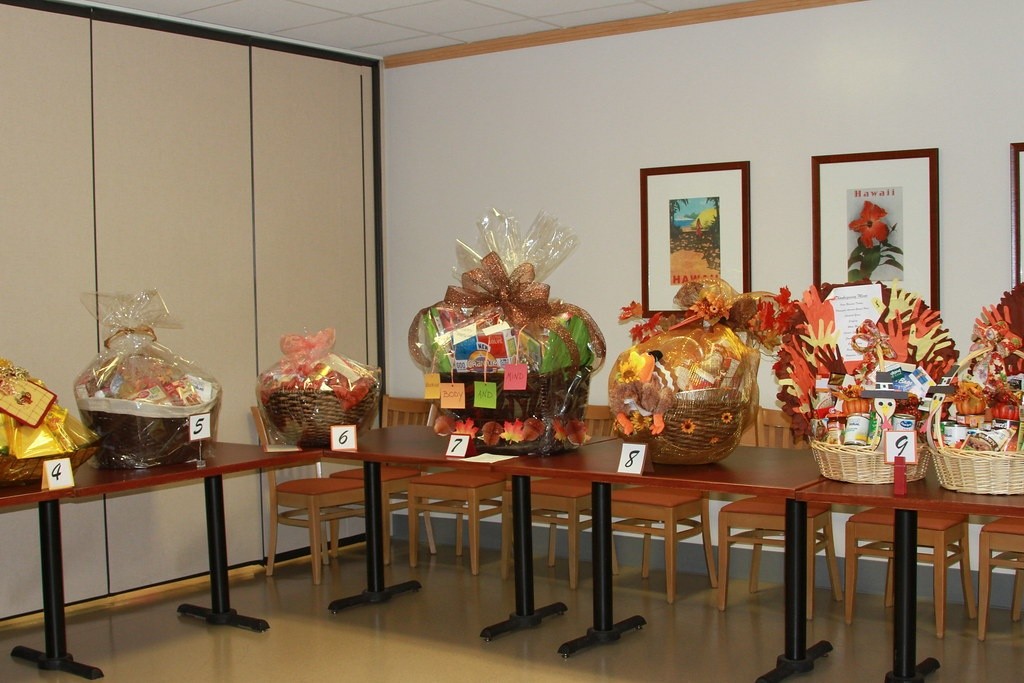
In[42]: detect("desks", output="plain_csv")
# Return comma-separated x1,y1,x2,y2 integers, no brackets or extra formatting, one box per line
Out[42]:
0,443,323,679
321,424,619,643
492,438,833,682
796,479,1024,683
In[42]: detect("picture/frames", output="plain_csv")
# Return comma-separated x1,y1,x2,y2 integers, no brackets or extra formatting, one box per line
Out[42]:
640,161,751,319
812,147,941,317
1007,142,1024,289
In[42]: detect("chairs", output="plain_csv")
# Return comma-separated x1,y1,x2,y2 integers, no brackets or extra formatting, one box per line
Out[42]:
330,394,1024,642
251,406,392,585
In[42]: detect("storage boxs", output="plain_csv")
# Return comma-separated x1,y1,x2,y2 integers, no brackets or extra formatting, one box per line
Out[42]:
687,355,744,391
439,314,592,376
77,375,212,409
